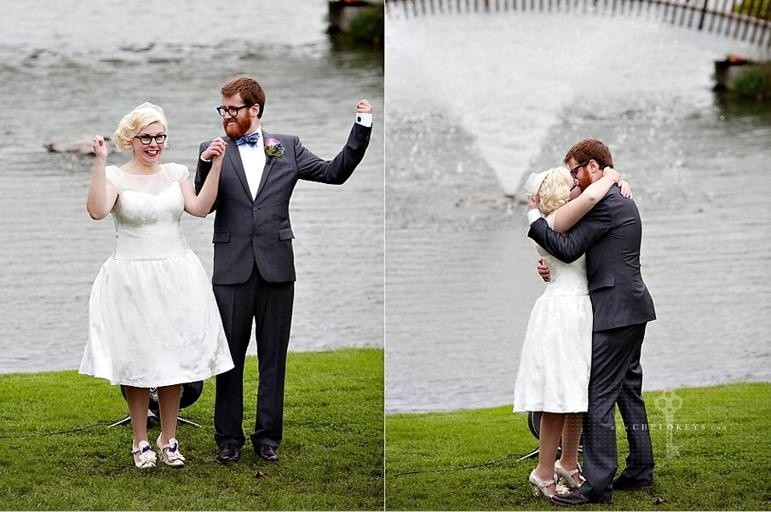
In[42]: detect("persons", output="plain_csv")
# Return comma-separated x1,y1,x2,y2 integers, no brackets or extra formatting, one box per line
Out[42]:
195,77,373,462
526,139,656,508
78,102,235,469
513,166,633,498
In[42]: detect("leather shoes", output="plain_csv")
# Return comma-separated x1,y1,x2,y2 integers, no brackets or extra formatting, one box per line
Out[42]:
551,486,611,506
613,474,653,490
219,443,240,461
253,444,279,461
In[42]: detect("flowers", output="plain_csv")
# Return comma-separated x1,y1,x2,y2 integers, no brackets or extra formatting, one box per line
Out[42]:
264,137,286,159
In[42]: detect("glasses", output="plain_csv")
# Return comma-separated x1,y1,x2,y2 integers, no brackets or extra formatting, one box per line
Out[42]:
217,105,252,117
570,172,581,192
134,135,166,145
571,161,589,175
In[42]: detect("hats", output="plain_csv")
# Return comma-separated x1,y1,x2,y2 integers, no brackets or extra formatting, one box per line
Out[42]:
522,168,554,202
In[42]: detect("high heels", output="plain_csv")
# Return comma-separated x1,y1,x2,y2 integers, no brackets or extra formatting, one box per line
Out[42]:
528,468,571,500
156,432,185,467
552,458,587,491
131,439,157,469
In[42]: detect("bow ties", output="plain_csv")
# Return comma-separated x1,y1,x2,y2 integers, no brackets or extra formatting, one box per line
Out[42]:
234,133,258,146
